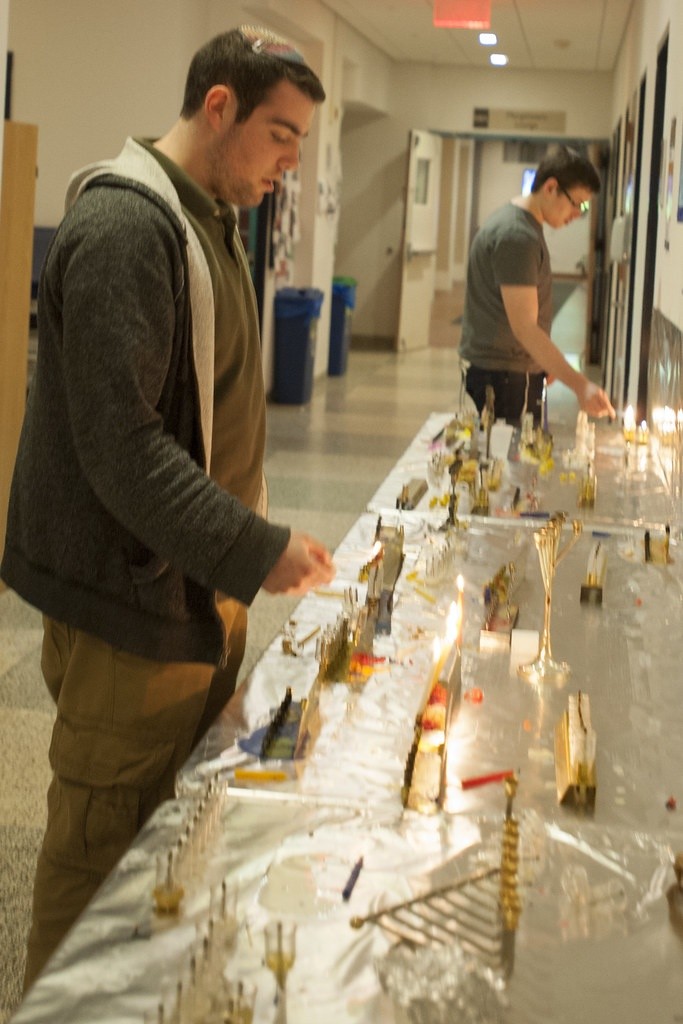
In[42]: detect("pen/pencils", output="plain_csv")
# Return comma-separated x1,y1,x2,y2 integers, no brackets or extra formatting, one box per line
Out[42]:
341,854,363,896
460,767,521,790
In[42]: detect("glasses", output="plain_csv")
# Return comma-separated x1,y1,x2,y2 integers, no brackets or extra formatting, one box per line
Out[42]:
561,187,583,210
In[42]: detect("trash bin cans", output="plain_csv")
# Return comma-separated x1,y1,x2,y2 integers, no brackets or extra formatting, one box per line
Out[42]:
328,275,357,377
270,286,323,406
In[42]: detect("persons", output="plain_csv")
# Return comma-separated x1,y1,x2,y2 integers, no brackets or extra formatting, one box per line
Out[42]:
458,153,617,433
1,24,336,997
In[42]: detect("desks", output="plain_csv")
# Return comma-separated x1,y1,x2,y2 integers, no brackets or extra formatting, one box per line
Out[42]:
9,410,683,1024
